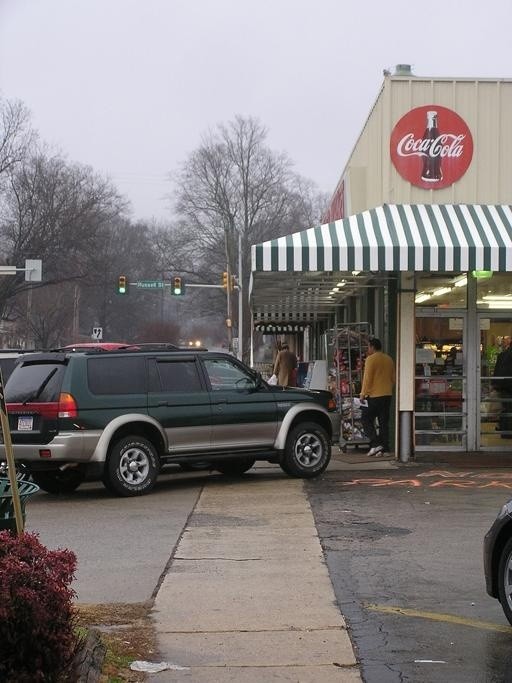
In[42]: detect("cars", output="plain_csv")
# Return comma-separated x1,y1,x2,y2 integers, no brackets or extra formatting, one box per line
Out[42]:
221,336,227,348
177,337,201,346
482,500,512,628
0,343,139,388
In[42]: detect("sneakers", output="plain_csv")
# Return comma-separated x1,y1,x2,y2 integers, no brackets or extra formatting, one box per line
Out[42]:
365,446,385,458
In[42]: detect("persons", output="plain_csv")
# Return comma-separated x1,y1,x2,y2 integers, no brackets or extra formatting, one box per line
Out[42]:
441,347,463,376
491,340,512,440
357,337,396,460
272,342,300,387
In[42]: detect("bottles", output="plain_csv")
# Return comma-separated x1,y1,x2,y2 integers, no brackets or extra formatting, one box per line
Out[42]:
420,111,445,182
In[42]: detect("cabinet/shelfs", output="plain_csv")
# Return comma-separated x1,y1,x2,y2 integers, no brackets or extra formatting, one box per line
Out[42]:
325,322,373,453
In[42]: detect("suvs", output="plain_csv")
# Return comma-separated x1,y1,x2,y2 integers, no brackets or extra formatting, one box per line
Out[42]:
0,343,340,497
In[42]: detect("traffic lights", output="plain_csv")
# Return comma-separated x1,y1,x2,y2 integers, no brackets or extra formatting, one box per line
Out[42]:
226,320,231,328
174,277,181,295
223,273,227,288
118,277,125,293
